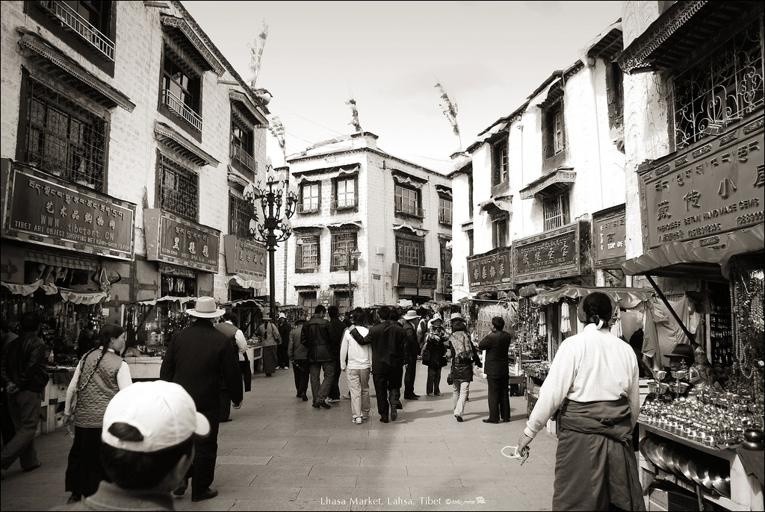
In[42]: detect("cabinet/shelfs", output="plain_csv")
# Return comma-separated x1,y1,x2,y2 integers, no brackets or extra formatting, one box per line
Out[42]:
126,357,162,379
525,365,550,422
238,346,264,374
637,392,765,512
40,374,72,434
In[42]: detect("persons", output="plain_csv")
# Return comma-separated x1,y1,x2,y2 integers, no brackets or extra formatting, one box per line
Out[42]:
518,291,647,512
659,344,699,405
1,296,512,510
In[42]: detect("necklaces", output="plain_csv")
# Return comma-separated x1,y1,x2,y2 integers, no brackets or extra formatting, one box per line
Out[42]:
729,267,764,415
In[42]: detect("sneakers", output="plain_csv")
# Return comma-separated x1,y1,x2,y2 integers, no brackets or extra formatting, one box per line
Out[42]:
351,416,363,424
275,366,281,370
284,366,289,370
361,410,369,420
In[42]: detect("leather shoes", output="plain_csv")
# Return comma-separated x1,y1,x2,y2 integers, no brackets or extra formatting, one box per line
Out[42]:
380,417,388,423
404,391,420,400
328,398,340,403
500,417,511,422
191,488,218,502
313,402,321,408
484,417,501,424
454,414,463,422
319,402,331,409
390,404,397,421
170,478,189,498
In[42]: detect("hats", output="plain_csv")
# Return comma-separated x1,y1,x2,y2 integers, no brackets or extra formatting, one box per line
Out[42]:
186,296,227,319
101,380,212,454
450,312,462,320
430,313,443,324
295,319,305,323
403,310,422,320
261,315,273,320
279,313,287,319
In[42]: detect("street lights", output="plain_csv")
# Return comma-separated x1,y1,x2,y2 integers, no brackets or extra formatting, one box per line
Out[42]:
243,164,298,320
333,247,362,310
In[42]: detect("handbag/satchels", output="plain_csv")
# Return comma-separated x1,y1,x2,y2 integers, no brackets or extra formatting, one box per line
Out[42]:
263,332,267,339
439,357,448,367
463,365,474,379
422,349,435,365
71,391,78,414
275,338,280,343
447,374,453,385
